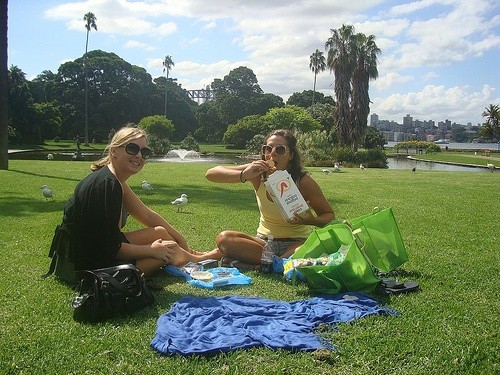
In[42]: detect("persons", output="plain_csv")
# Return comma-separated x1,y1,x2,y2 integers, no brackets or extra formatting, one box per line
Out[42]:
40,126,223,293
206,128,335,274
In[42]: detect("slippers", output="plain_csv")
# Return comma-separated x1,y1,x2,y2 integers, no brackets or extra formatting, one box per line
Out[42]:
376,280,420,295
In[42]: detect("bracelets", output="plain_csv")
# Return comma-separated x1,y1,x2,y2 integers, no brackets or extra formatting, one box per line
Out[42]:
240,169,247,184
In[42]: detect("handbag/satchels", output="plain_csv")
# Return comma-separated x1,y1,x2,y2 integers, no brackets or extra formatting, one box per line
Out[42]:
291,224,378,295
351,206,409,273
74,264,154,321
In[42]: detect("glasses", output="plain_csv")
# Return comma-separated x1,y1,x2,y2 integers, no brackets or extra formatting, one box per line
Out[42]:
118,142,152,159
262,145,290,156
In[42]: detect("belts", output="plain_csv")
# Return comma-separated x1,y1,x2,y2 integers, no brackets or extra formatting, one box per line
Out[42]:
261,237,298,241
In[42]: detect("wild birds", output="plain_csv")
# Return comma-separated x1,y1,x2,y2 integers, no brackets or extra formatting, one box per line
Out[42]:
412,167,417,173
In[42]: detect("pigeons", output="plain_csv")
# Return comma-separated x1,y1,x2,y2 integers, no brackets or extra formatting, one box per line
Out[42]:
172,194,188,212
48,154,54,159
142,181,153,193
42,185,54,200
334,164,338,169
360,164,364,170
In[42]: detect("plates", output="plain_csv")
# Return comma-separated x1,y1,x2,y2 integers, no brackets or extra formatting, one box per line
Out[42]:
191,271,214,279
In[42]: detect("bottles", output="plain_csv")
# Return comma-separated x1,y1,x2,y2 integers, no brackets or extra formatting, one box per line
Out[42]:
260,234,276,274
